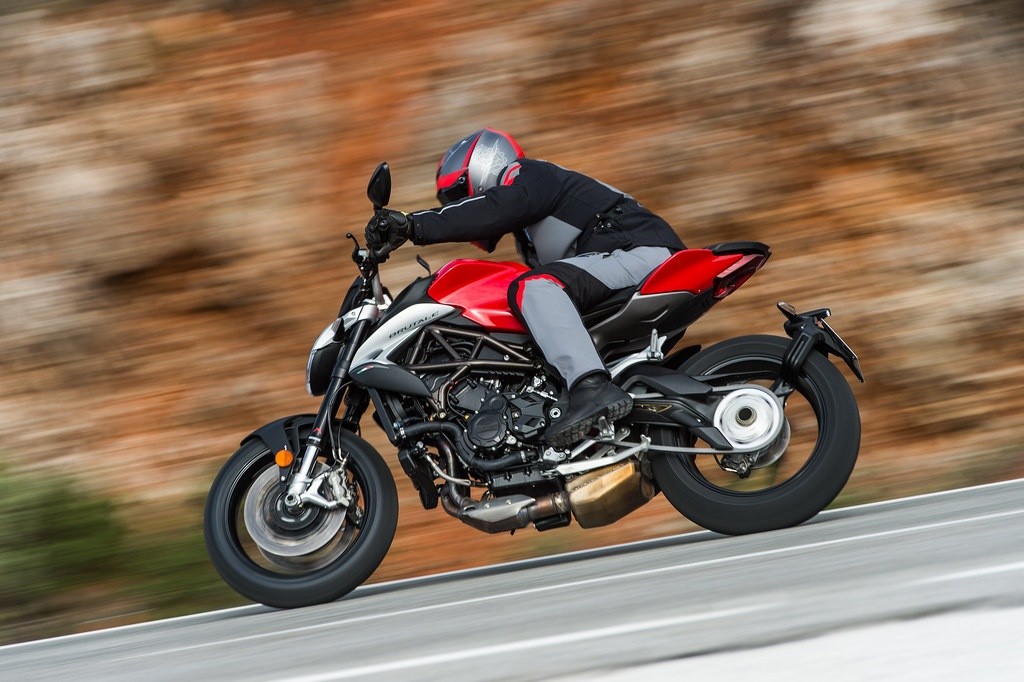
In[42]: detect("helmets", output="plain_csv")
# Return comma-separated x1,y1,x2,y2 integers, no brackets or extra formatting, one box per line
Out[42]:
433,127,526,254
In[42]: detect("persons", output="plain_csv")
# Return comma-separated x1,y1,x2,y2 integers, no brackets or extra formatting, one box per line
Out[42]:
365,127,687,446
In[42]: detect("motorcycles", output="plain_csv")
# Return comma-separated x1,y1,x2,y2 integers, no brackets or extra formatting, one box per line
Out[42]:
201,159,866,611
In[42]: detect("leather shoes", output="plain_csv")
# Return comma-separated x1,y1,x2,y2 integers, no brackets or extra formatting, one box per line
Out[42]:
543,372,634,447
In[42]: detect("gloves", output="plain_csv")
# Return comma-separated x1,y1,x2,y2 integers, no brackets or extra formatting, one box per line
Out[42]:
364,206,414,259
